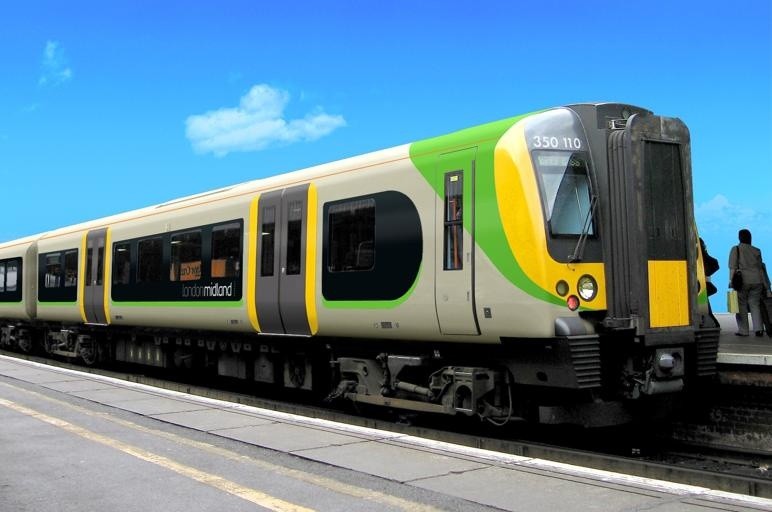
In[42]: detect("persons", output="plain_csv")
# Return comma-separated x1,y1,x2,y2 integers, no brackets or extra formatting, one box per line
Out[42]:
699,237,720,328
728,229,771,337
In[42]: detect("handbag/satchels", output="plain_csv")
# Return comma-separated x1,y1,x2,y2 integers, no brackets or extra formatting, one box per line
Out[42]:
707,282,717,296
727,290,740,313
704,254,719,276
732,271,742,292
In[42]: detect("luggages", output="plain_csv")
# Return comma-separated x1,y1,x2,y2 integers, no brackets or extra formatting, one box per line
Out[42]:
760,294,772,337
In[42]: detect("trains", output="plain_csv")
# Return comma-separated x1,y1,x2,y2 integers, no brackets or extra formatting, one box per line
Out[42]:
0,101,722,446
